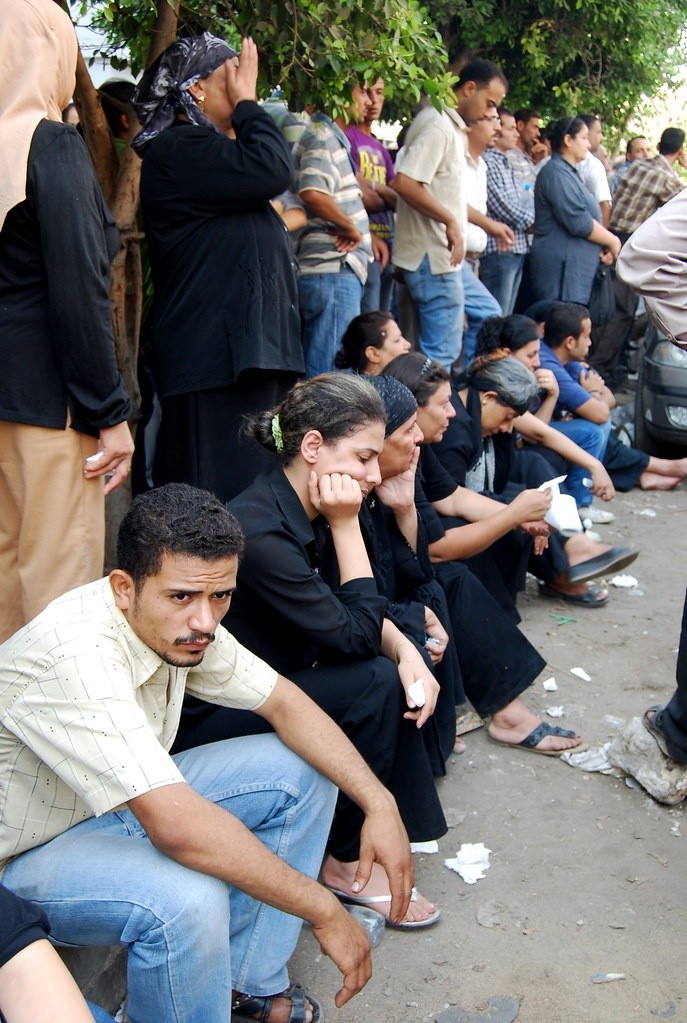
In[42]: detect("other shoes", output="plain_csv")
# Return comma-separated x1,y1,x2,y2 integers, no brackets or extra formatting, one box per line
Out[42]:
642,704,673,759
567,545,642,583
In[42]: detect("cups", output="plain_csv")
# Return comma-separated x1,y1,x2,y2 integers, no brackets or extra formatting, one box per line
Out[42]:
303,901,386,947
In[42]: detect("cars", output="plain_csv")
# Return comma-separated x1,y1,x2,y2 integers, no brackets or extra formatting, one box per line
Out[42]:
634,323,687,460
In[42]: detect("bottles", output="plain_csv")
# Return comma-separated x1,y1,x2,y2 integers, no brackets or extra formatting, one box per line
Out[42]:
519,182,535,213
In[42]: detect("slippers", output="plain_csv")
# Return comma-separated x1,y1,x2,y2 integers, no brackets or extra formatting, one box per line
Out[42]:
323,882,441,926
488,720,587,755
538,580,610,608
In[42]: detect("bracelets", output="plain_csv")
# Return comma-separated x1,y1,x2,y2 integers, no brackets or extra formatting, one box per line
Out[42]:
393,639,411,664
589,389,602,395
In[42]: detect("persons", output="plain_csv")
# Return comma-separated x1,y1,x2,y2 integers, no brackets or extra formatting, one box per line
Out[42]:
0,0,687,1023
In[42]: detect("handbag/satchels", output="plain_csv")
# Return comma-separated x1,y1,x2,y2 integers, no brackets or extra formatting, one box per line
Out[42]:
590,253,616,326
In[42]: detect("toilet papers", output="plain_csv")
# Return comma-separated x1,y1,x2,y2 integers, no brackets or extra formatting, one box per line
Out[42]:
535,474,583,537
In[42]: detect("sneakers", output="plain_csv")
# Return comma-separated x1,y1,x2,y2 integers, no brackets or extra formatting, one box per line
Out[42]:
578,505,615,524
585,530,602,542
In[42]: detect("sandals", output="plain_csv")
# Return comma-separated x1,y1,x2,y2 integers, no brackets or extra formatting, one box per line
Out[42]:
232,983,324,1023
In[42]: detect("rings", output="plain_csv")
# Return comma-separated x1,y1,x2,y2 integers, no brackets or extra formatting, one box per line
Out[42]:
350,241,354,245
